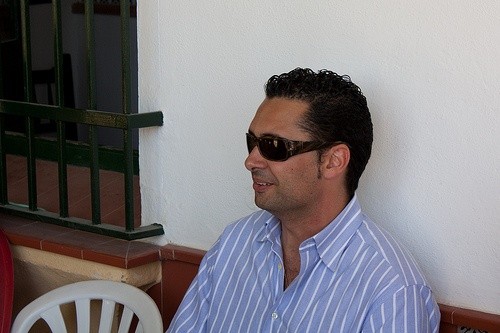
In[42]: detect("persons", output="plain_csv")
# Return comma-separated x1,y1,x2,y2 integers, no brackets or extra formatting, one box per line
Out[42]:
164,67,443,333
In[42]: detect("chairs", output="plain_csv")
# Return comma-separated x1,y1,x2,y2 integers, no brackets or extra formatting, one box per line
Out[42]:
0,230,163,333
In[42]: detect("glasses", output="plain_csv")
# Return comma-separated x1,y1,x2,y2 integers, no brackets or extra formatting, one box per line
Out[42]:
245,132,333,162
